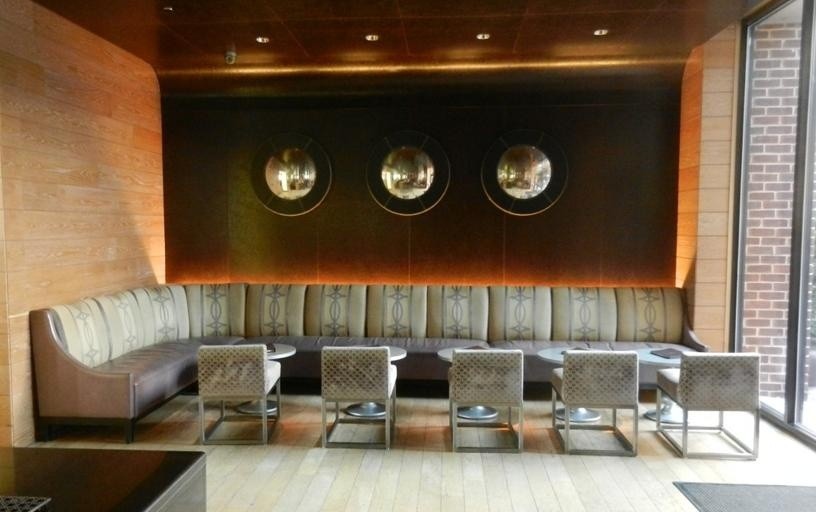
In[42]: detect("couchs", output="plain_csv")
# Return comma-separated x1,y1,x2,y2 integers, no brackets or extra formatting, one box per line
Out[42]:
31,282,708,445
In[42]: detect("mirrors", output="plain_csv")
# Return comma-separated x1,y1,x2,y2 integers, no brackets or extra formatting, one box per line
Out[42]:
249,130,333,220
364,130,451,219
480,129,570,219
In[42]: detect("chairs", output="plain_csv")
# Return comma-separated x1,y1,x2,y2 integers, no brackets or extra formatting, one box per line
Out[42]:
550,349,640,458
195,342,283,446
447,349,525,454
655,351,761,462
320,342,399,449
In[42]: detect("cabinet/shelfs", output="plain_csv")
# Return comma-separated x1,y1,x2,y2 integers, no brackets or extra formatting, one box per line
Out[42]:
0,447,207,512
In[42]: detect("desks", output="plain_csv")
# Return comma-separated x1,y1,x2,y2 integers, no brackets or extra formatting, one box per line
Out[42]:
635,348,695,425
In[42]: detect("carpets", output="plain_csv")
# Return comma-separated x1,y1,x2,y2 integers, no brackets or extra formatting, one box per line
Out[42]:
673,480,816,512
